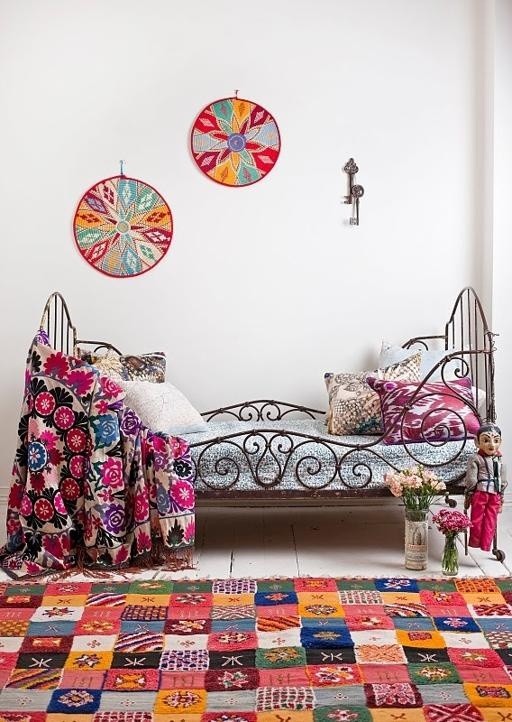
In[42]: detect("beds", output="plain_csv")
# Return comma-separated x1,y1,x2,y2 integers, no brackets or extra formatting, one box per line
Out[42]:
29,286,506,581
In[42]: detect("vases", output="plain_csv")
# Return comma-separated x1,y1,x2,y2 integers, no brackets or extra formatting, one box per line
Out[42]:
404,516,430,572
442,537,463,577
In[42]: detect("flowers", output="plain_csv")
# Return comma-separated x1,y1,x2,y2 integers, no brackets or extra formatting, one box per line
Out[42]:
433,506,475,574
382,463,451,520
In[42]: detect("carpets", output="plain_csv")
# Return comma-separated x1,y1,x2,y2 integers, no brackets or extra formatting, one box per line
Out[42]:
0,577,511,721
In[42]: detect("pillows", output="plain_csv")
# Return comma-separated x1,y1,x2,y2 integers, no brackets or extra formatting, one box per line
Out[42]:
365,374,483,446
113,378,209,437
73,344,167,384
324,350,421,437
376,339,475,381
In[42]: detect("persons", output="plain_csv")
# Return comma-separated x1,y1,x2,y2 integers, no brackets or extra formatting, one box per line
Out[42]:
464,424,505,551
